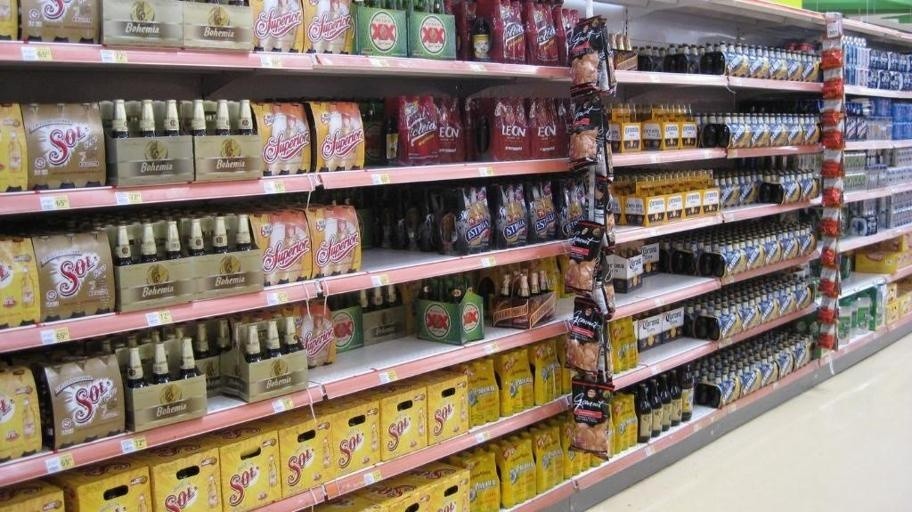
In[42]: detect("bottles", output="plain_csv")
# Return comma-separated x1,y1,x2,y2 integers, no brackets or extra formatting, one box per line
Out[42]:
115,96,553,186
840,35,911,344
115,261,550,431
111,183,550,311
468,13,491,59
614,38,821,455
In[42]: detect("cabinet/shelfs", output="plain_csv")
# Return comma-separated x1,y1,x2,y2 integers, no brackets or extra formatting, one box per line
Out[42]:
0,0,912,512
